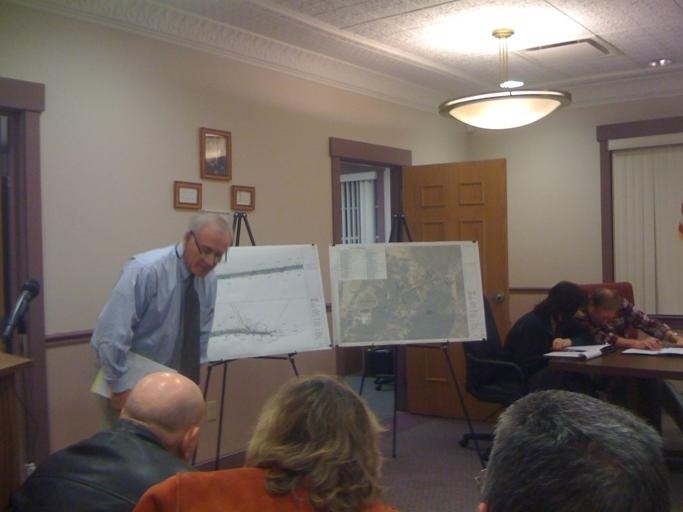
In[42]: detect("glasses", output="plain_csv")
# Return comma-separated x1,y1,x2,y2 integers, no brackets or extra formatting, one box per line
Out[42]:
189,229,228,269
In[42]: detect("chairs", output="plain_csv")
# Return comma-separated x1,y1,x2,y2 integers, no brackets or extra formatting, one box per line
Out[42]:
459,292,528,461
564,282,638,343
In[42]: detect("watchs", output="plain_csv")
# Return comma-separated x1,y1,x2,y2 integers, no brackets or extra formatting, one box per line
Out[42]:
668,332,678,342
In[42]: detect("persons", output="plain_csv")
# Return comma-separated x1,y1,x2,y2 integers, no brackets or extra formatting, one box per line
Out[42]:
131,373,398,511
503,281,592,398
92,216,233,467
479,388,671,512
8,373,206,511
576,287,682,438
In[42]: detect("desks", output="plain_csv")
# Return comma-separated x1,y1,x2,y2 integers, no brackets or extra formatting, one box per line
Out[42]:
0,348,34,512
549,329,683,425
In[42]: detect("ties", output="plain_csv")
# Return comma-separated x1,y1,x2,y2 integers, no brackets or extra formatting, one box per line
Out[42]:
178,273,201,388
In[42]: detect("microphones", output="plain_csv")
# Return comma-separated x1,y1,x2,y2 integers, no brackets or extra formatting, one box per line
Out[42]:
3,278,41,339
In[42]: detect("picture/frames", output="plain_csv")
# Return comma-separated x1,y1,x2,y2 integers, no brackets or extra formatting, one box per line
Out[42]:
200,127,233,181
231,185,256,211
173,181,202,209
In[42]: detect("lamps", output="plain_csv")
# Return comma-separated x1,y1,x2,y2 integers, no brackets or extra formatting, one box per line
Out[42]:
440,29,572,129
